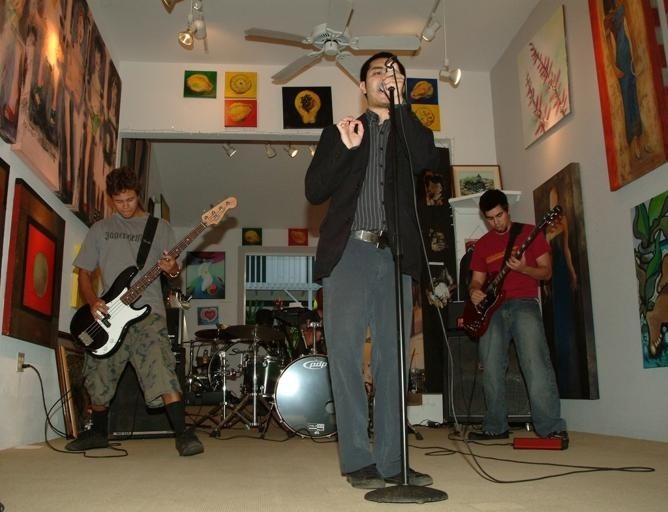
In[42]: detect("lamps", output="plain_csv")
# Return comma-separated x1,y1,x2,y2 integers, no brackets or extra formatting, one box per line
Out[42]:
420,0,461,86
222,142,317,158
177,0,207,46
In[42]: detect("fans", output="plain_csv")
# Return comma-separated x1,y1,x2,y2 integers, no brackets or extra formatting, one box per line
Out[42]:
242,1,420,86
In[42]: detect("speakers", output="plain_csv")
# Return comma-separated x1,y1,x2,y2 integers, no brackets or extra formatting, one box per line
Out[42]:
108,350,186,440
426,300,542,424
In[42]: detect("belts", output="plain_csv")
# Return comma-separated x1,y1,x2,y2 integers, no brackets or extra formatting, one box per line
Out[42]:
350,228,390,249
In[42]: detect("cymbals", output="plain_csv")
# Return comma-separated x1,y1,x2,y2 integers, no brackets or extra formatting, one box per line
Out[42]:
195,328,224,339
223,325,256,339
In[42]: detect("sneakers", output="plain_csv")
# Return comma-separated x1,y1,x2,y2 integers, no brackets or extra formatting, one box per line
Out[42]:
175,427,204,456
65,429,108,451
384,468,433,487
469,429,509,440
548,430,569,450
347,464,386,489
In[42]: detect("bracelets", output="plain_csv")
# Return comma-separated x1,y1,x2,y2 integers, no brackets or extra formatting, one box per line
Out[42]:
170,265,181,278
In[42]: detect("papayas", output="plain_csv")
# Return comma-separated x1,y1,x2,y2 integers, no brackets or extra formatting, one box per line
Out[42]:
186,73,213,92
228,103,252,121
410,81,433,99
295,90,321,124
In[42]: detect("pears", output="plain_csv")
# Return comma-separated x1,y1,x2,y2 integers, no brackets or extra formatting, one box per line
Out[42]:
289,230,306,243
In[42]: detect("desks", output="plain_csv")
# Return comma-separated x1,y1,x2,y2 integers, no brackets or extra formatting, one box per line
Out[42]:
442,299,534,432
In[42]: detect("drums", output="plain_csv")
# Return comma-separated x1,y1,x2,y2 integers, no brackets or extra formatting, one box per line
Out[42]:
208,340,269,397
244,354,283,397
274,354,337,438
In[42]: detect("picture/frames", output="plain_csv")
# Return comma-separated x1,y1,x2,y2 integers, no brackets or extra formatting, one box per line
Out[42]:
55,330,85,440
451,165,503,198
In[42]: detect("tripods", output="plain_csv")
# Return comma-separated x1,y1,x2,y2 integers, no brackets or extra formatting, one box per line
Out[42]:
214,333,294,438
191,347,251,436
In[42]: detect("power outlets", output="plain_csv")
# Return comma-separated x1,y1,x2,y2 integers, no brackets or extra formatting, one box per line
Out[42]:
18,353,24,373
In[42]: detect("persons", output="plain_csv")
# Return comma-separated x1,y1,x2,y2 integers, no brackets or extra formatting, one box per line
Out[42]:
543,188,583,399
90,39,104,113
65,165,203,456
108,76,119,125
603,1,654,158
291,286,326,362
66,8,86,100
468,189,570,450
303,53,434,493
294,320,323,358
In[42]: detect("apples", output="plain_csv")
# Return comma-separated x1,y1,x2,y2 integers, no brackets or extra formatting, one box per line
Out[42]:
245,230,258,243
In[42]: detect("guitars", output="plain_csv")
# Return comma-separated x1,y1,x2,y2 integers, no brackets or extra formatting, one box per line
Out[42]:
456,205,562,337
70,197,237,358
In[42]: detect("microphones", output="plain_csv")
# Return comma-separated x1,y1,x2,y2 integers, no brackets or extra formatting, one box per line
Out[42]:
379,69,398,92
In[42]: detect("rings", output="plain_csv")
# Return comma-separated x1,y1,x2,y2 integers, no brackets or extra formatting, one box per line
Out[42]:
100,303,104,307
